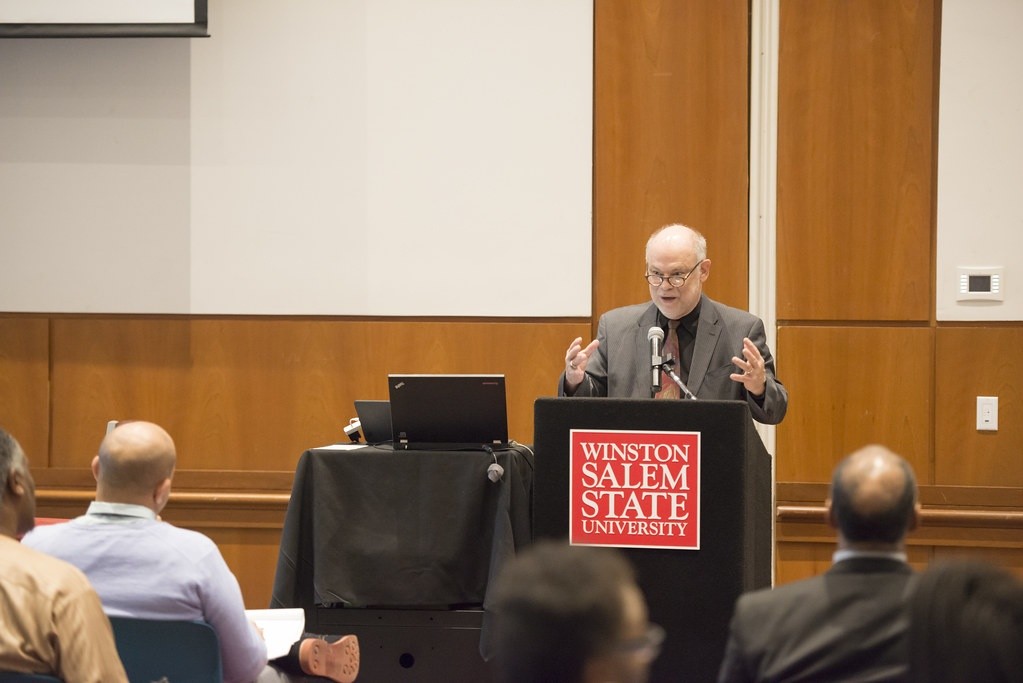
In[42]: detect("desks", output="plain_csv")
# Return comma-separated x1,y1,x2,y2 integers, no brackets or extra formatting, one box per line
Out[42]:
309,444,534,609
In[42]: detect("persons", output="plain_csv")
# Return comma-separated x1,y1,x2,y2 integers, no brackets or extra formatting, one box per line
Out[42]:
557,223,790,426
490,538,664,683
0,428,128,683
19,419,360,683
908,554,1023,683
717,444,923,683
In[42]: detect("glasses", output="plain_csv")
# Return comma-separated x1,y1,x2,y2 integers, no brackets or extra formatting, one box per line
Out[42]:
645,259,703,288
605,624,665,662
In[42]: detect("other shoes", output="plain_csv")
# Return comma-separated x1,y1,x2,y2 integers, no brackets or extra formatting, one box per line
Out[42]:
271,634,360,683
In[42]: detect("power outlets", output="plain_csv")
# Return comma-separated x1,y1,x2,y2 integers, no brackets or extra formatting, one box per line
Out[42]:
975,396,998,431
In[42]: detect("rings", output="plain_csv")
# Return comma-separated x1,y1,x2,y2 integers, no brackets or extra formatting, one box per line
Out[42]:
745,368,753,374
570,360,576,369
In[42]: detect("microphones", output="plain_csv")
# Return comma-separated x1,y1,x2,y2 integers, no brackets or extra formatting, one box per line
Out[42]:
647,326,664,393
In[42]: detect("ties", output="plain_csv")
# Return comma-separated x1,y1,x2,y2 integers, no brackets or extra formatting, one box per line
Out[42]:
655,320,680,399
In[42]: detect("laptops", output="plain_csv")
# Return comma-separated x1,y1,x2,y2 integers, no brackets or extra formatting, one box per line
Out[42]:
388,373,515,451
354,399,394,445
